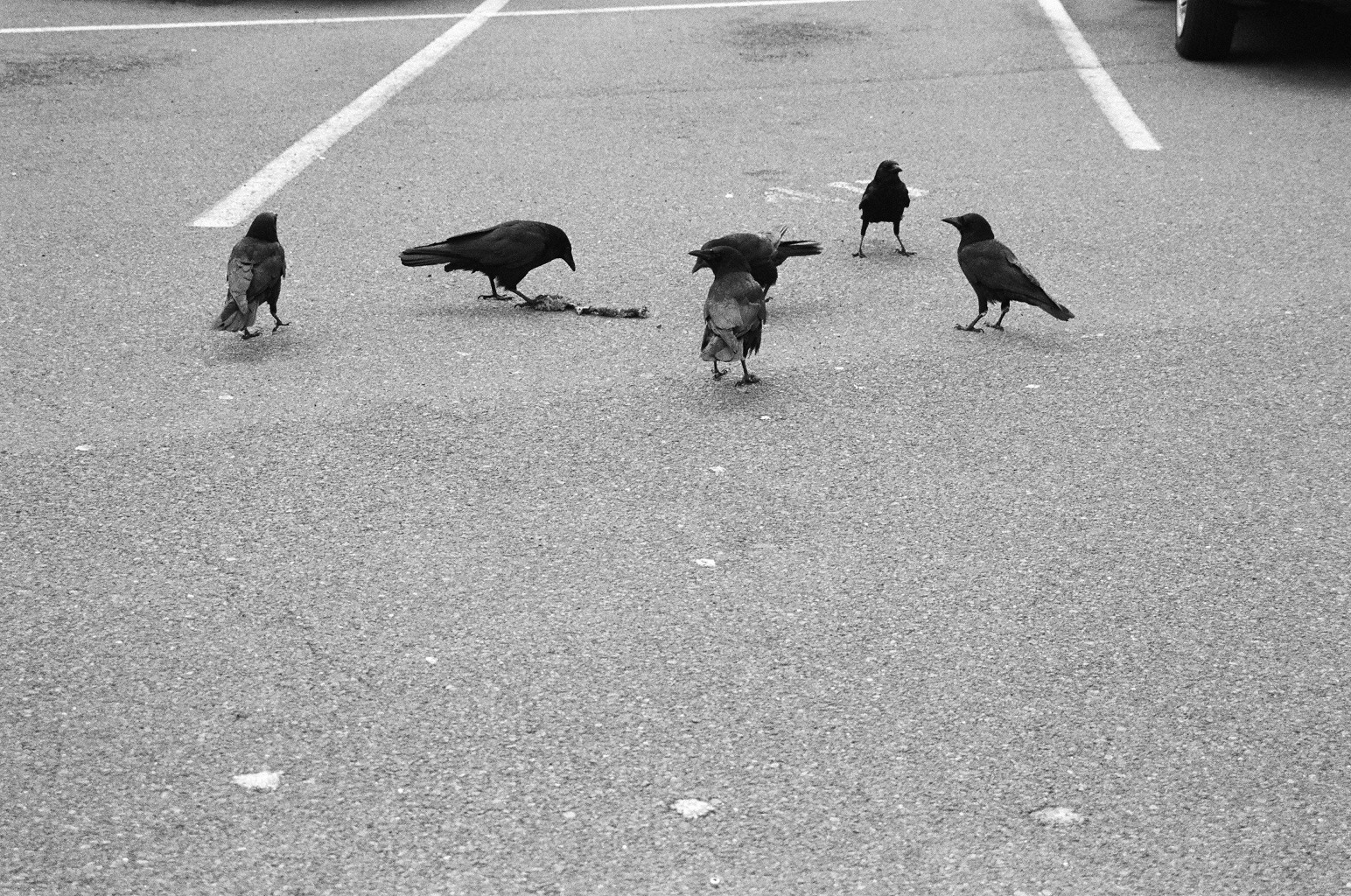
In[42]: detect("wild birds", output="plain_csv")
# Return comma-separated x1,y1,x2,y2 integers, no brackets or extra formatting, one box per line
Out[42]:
851,160,920,258
941,212,1074,334
209,211,293,341
691,224,827,302
689,246,771,386
400,220,574,309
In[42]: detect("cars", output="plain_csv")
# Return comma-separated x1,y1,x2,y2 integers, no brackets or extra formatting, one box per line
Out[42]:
1172,0,1351,64
